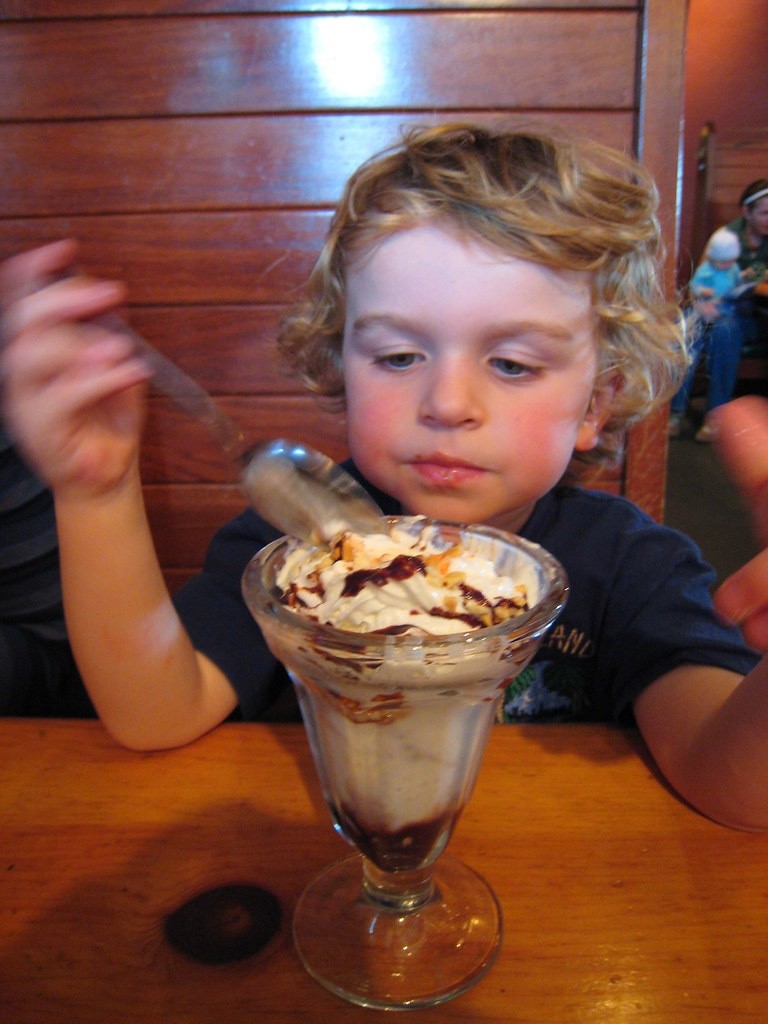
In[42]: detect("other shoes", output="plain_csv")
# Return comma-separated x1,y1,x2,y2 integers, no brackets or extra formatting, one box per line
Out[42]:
694,421,715,444
666,414,682,438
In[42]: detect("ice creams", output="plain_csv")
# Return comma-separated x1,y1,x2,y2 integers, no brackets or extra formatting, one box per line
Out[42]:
265,526,540,872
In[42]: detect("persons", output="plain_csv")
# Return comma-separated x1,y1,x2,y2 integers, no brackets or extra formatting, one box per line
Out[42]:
1,121,768,834
667,177,768,548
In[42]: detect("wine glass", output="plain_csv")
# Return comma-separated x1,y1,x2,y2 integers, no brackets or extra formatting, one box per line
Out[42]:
240,515,569,1009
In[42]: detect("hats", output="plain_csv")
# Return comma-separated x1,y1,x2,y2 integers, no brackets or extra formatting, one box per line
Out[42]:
704,226,741,263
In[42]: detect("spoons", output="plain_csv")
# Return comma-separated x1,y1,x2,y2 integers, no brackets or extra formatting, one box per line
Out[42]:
97,304,386,548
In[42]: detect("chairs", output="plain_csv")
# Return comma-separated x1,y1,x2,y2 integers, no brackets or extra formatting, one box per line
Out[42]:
680,120,768,394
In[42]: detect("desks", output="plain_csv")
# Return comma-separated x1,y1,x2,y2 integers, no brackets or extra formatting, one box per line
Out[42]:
0,716,768,1024
